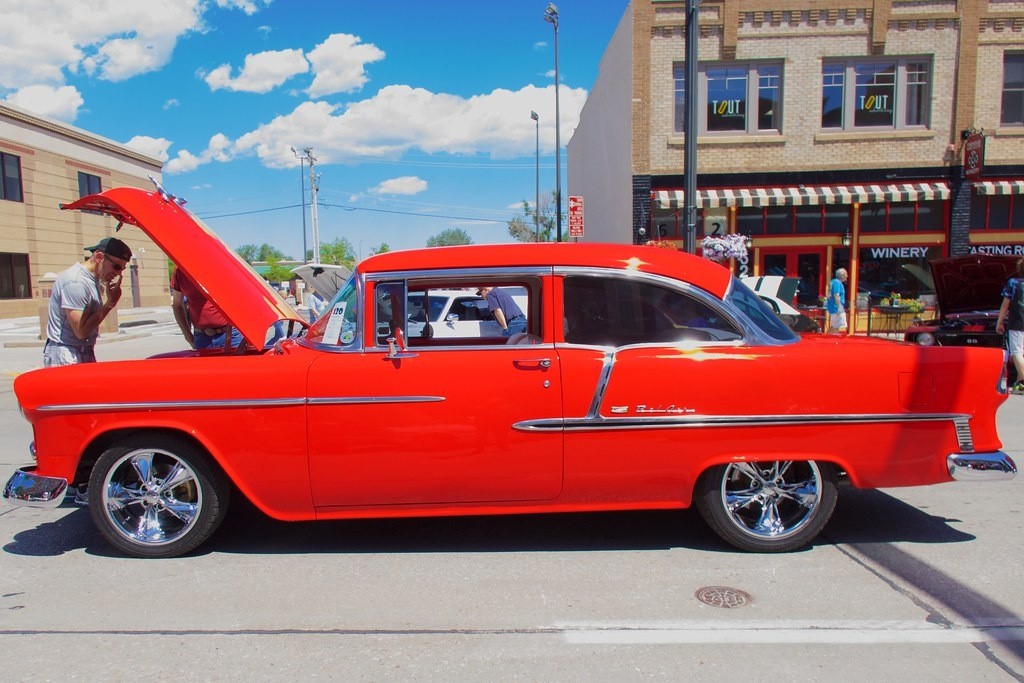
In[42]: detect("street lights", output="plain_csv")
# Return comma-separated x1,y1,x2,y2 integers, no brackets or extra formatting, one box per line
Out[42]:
544,2,562,244
530,110,540,242
291,147,314,264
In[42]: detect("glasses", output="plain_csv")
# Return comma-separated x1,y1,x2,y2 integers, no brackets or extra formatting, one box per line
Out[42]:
96,250,126,271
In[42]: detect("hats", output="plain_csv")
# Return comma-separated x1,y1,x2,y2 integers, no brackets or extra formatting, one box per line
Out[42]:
83,237,132,262
475,287,488,296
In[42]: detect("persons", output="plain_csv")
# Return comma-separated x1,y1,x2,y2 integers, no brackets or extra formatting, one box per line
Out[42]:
41,237,132,505
476,286,528,336
171,264,242,350
995,258,1024,394
308,289,329,326
266,289,288,346
826,268,848,333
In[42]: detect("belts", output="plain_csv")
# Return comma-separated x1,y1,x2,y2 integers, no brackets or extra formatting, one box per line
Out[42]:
194,326,227,336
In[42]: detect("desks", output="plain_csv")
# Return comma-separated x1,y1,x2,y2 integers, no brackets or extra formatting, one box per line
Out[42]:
874,304,924,341
817,306,830,333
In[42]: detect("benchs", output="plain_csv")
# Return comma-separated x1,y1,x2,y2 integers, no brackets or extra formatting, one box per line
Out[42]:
650,327,712,345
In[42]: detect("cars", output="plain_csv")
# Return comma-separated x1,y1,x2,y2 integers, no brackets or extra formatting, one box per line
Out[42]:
3,175,1017,562
904,252,1024,387
739,275,823,333
290,264,528,338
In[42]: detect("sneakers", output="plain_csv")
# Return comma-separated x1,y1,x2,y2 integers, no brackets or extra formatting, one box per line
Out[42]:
62,483,88,506
1010,381,1024,394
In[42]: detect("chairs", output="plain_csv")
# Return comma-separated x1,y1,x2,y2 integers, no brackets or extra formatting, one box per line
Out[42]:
458,306,482,321
907,301,938,326
505,333,532,345
800,305,827,334
515,334,542,345
